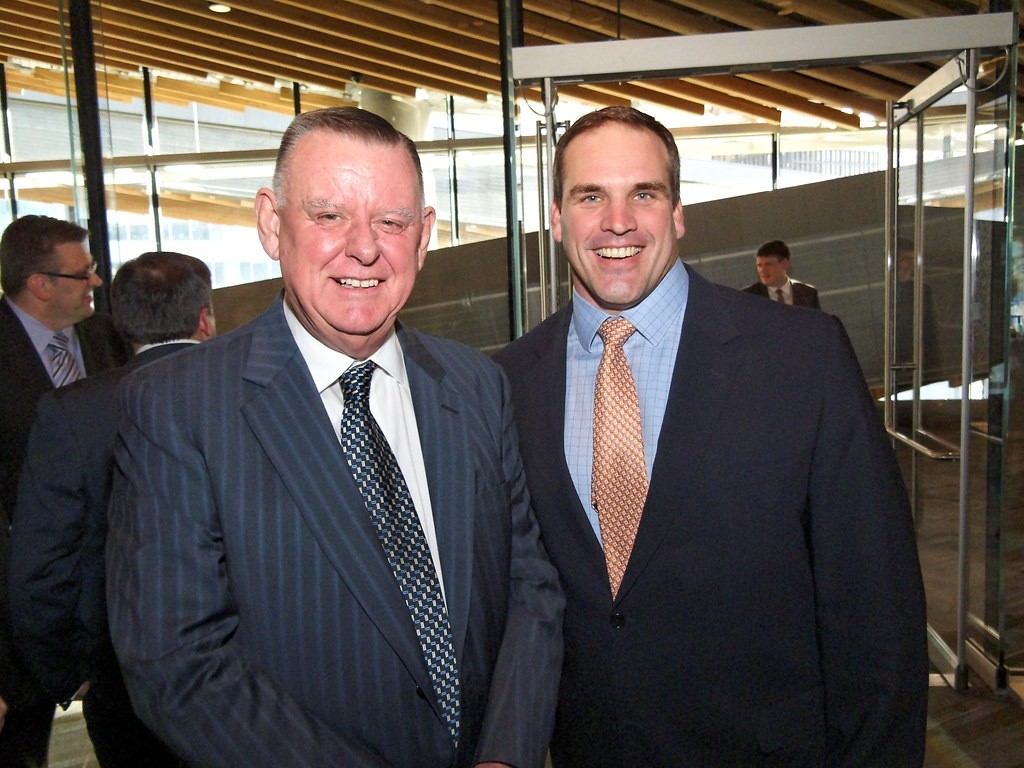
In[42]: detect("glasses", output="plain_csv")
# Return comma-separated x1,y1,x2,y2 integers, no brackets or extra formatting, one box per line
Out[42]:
21,260,98,287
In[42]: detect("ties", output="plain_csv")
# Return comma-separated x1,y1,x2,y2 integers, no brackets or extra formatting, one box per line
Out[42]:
591,318,649,601
47,332,83,390
337,361,459,750
775,288,786,305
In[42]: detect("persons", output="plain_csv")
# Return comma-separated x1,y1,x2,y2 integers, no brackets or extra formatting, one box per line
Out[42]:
108,107,565,766
0,214,102,768
19,252,218,768
733,241,817,318
495,105,924,767
